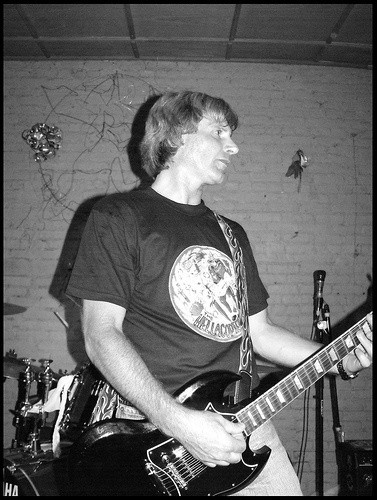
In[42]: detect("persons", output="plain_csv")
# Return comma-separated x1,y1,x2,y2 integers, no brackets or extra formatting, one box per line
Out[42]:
64,89,374,499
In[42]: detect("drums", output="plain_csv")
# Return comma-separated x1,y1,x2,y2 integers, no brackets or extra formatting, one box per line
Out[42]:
4,449,57,497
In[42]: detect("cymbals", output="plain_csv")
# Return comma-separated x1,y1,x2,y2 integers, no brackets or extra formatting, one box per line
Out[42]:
3,358,39,380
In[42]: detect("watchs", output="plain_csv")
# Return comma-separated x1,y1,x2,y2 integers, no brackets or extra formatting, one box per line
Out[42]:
336,359,360,381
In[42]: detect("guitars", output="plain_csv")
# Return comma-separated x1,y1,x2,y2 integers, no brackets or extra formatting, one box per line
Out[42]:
71,311,373,500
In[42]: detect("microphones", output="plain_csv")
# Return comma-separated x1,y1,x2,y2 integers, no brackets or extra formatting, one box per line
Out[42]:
313,269,327,326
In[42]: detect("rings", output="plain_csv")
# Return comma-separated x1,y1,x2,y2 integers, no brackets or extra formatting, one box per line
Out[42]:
223,453,229,461
